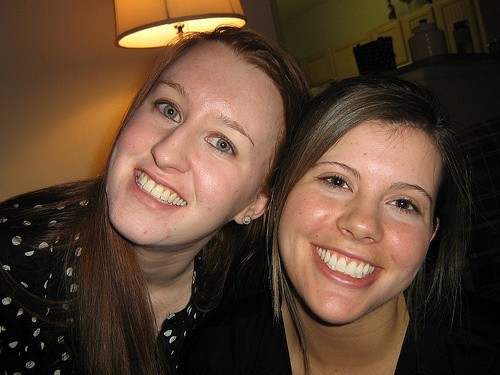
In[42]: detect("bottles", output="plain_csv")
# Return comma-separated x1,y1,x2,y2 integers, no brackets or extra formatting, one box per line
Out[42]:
452,20,476,54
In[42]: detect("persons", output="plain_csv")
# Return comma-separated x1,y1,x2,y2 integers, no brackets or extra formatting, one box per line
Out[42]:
181,76,500,375
0,23,313,374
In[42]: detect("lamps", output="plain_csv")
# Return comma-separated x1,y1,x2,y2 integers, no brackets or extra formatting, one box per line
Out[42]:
113,0,248,49
